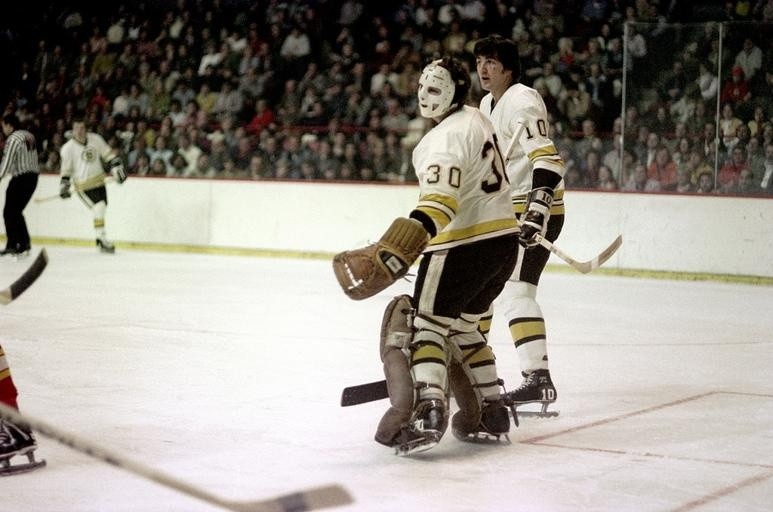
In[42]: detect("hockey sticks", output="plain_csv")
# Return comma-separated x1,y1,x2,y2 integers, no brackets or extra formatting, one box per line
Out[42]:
0,403,356,512
0,248,48,305
516,219,622,272
341,380,388,407
33,178,115,204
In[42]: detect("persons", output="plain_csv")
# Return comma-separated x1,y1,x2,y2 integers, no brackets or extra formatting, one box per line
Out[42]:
333,58,521,450
60,118,127,252
0,114,41,255
1,341,37,460
470,34,564,403
1,1,773,198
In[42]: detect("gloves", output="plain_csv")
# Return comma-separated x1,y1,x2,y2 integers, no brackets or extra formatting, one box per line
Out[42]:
110,158,126,183
519,186,553,249
59,177,71,198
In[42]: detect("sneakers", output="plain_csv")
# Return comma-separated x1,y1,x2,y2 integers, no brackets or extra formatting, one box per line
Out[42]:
475,403,509,433
394,400,444,446
97,238,114,253
0,423,37,458
0,242,30,256
503,370,557,402
